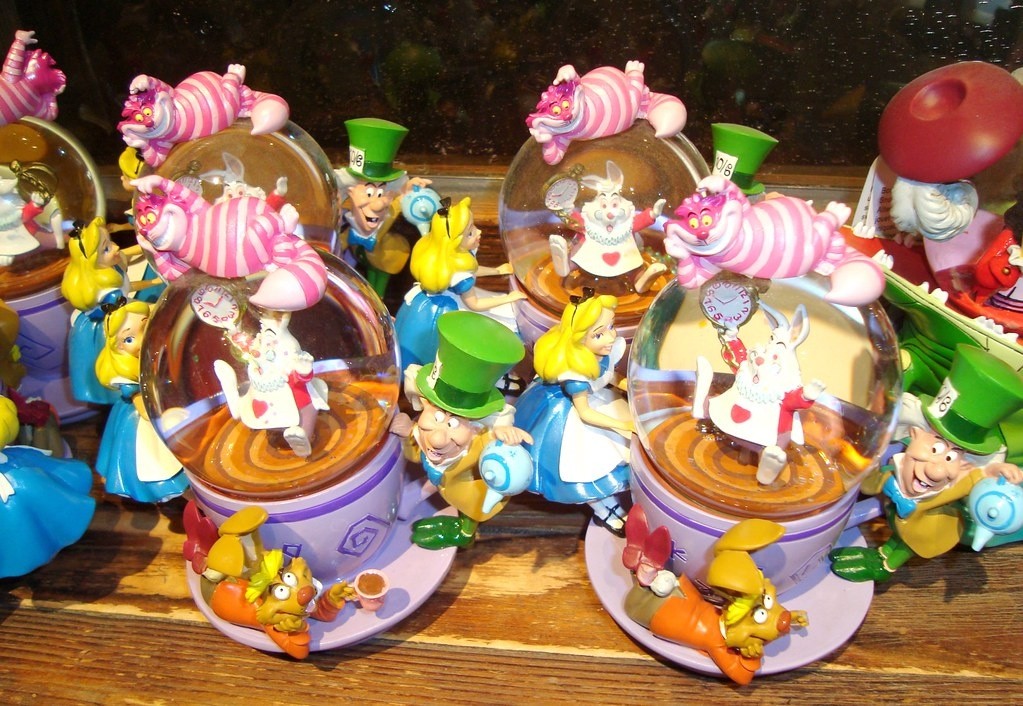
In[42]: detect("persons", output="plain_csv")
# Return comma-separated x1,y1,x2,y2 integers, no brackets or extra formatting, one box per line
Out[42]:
390,311,535,550
61,222,163,405
105,146,149,257
333,116,435,298
95,296,195,510
828,342,1023,583
0,301,97,578
396,197,529,380
514,288,640,534
